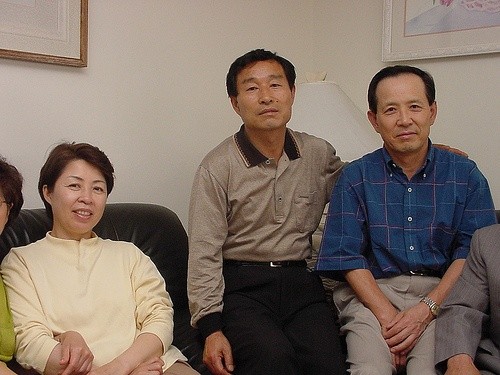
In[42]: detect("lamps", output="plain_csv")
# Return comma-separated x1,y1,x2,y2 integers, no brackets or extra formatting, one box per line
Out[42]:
289,81,383,253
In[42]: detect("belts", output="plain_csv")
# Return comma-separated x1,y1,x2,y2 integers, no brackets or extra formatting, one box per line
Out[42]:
406,269,441,277
229,259,306,268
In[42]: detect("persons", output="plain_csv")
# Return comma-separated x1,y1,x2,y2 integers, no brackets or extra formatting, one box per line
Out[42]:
433,223,500,375
0,153,94,375
187,48,469,375
314,66,500,375
1,141,201,375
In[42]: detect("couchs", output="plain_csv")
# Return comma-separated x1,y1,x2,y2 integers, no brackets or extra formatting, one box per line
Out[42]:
0,205,213,375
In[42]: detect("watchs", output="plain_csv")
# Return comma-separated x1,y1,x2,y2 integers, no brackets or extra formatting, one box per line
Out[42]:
420,297,440,316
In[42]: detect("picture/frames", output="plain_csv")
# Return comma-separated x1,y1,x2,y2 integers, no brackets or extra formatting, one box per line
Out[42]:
0,0,88,67
381,0,500,62
